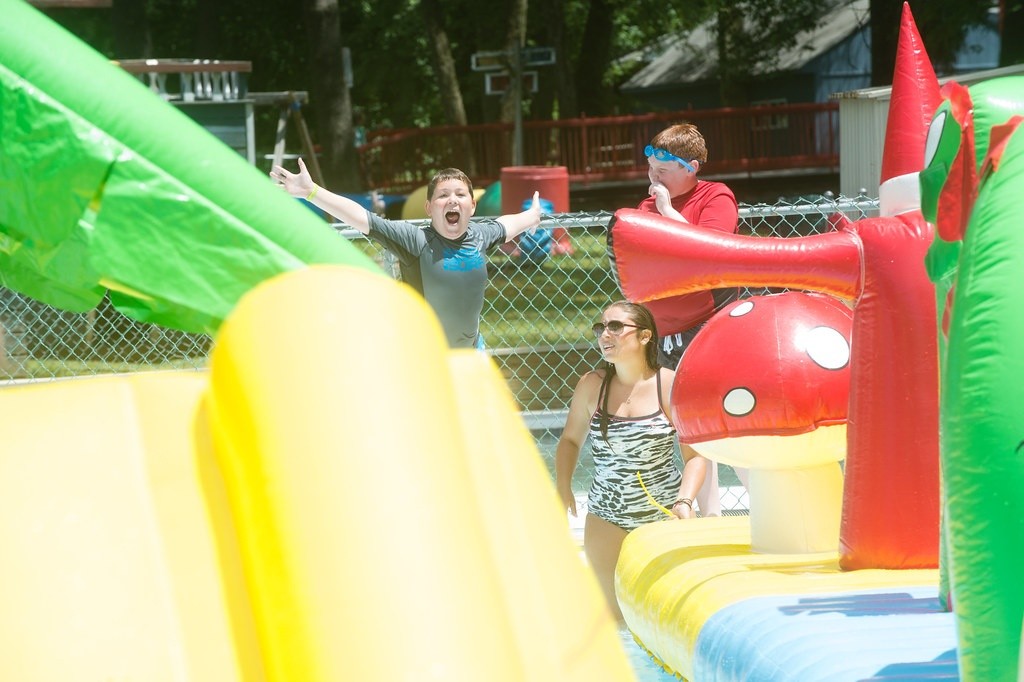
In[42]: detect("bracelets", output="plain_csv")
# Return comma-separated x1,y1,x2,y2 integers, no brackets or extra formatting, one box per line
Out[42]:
673,498,693,510
306,182,317,202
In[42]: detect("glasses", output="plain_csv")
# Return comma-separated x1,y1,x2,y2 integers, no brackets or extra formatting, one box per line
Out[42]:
592,320,646,338
644,146,696,173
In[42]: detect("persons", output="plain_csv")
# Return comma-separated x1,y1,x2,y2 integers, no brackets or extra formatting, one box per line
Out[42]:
270,158,541,350
630,122,741,371
554,299,706,619
